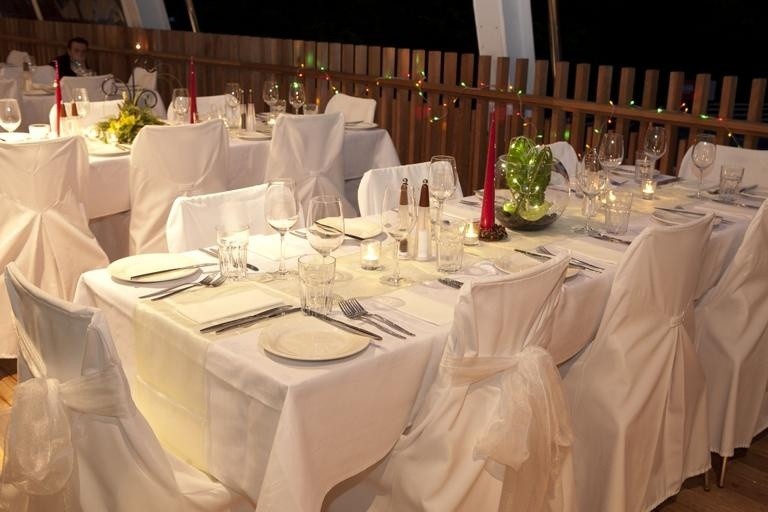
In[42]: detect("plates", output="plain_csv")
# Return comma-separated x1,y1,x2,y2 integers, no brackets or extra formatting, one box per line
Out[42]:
87,121,379,156
109,252,199,284
258,309,372,362
494,253,581,279
651,207,714,225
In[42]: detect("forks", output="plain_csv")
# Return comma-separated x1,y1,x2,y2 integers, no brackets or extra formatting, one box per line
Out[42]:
337,297,415,339
135,270,234,302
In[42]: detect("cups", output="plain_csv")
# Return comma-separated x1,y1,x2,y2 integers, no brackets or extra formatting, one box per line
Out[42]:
358,239,380,270
213,225,250,280
302,104,318,115
298,255,336,312
437,222,465,274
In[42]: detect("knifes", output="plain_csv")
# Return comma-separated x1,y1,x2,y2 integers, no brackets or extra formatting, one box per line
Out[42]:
197,245,261,272
200,304,302,335
513,248,586,270
302,307,384,341
129,261,219,280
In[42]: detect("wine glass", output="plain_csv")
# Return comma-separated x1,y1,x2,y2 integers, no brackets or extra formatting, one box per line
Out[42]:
306,195,345,261
265,177,298,280
486,129,716,241
378,181,418,288
0,55,305,138
427,154,458,215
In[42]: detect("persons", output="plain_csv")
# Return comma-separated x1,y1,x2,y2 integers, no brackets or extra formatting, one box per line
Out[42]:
50,37,89,79
